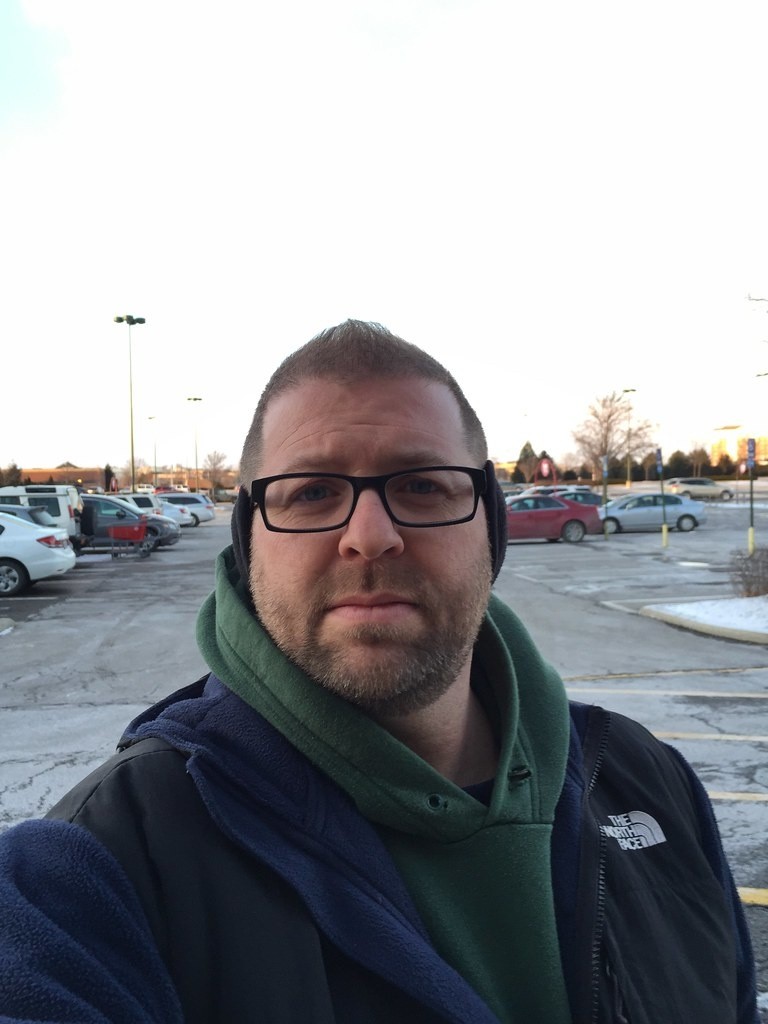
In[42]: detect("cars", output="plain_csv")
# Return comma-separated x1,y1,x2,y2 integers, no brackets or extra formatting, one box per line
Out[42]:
1,512,77,596
154,486,184,493
665,477,734,501
505,496,604,544
549,490,612,508
79,493,182,553
173,485,191,492
598,492,708,535
1,503,57,527
110,494,163,516
159,500,192,526
156,493,214,527
120,488,131,494
503,485,591,504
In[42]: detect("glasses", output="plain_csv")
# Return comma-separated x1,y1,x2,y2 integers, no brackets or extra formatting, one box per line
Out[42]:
243,462,489,534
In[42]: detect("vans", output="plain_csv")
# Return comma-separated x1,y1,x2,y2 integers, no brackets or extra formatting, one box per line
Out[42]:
0,485,85,543
131,484,156,493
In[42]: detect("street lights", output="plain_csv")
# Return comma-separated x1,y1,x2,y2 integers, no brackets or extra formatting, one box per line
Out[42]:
187,397,202,495
114,315,146,494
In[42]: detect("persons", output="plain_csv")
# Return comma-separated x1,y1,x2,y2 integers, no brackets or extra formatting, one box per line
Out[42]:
0,320,762,1024
